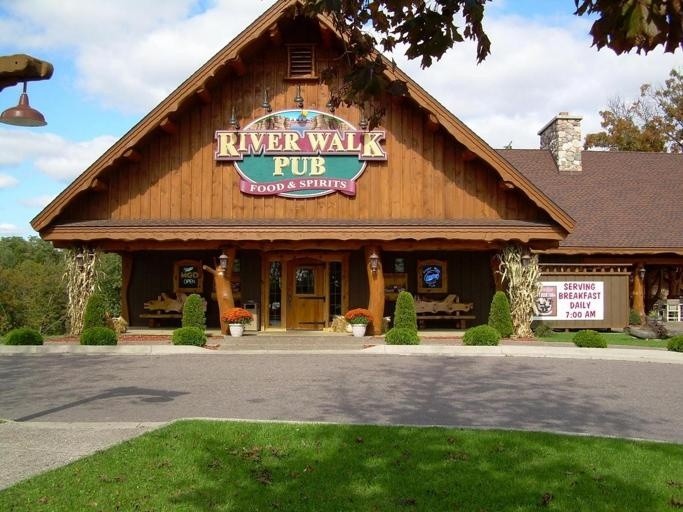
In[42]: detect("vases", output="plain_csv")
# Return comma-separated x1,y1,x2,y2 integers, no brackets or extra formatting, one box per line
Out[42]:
351,324,367,339
228,324,245,337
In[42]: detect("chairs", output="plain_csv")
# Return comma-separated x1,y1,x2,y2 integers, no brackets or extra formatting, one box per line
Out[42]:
662,298,681,322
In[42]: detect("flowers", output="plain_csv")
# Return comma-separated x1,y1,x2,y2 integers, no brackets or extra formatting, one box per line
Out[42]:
345,308,375,324
222,308,252,323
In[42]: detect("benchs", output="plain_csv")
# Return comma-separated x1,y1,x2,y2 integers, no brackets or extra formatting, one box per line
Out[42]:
140,292,209,328
408,294,477,330
